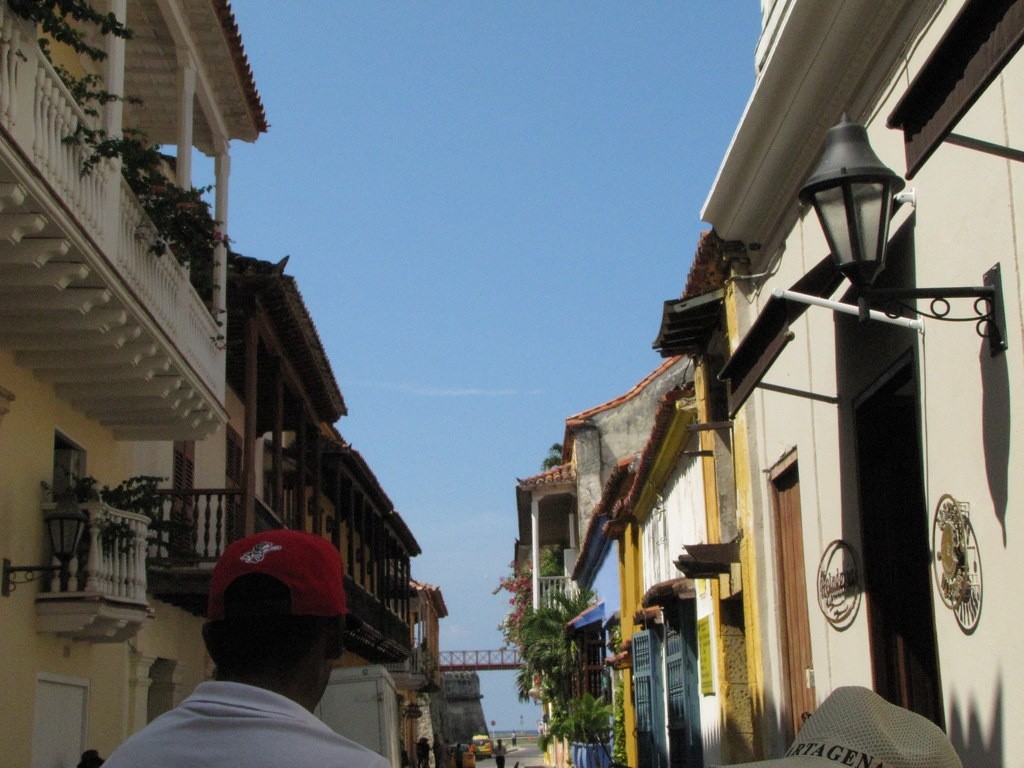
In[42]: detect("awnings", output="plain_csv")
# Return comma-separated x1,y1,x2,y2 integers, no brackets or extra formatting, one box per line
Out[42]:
561,601,607,630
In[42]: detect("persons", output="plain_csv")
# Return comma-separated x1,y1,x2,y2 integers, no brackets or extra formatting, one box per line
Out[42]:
495,739,506,768
512,730,517,747
442,738,452,768
432,734,441,768
396,737,410,768
101,528,395,768
416,737,431,768
454,743,463,768
724,683,964,768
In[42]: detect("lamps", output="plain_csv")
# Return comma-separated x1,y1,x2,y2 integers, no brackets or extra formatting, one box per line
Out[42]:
798,110,1012,357
1,486,88,602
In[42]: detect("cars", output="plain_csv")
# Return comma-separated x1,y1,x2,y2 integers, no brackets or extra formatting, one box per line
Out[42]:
447,744,476,768
470,736,493,759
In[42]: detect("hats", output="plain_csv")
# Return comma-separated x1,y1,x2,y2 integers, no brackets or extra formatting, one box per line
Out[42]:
707,685,964,767
207,531,352,624
